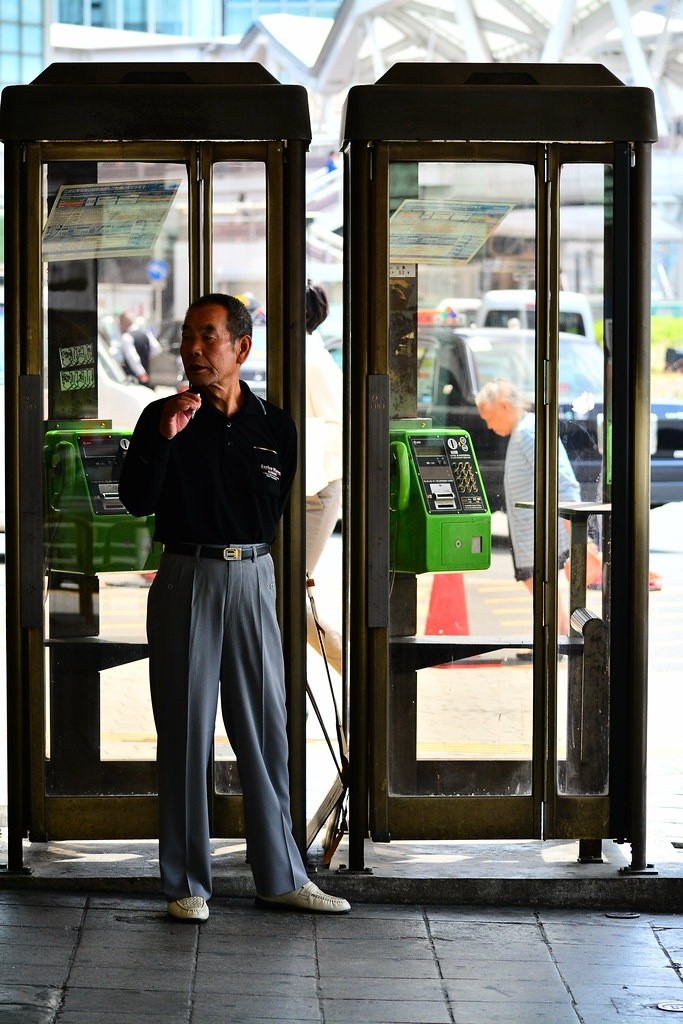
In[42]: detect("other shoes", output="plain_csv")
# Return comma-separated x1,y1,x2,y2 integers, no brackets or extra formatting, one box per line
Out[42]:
517,649,562,662
168,896,209,924
255,881,351,914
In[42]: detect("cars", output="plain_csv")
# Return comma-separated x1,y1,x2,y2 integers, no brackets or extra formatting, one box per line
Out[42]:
324,329,683,523
148,321,266,396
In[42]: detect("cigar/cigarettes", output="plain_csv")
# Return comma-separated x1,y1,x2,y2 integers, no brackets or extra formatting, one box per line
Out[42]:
190,393,201,419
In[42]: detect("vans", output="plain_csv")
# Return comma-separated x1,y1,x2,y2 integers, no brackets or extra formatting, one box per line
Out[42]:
475,289,594,341
436,298,480,329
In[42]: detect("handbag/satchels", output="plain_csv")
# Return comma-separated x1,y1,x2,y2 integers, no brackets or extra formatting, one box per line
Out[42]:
564,542,602,586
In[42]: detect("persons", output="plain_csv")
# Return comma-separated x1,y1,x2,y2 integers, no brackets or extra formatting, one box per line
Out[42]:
305,280,343,675
119,313,164,384
116,292,351,922
473,374,585,663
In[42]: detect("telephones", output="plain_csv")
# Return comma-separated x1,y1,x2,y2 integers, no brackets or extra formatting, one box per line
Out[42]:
388,427,493,575
41,428,163,574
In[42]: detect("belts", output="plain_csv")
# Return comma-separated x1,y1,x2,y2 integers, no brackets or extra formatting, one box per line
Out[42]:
163,543,270,561
